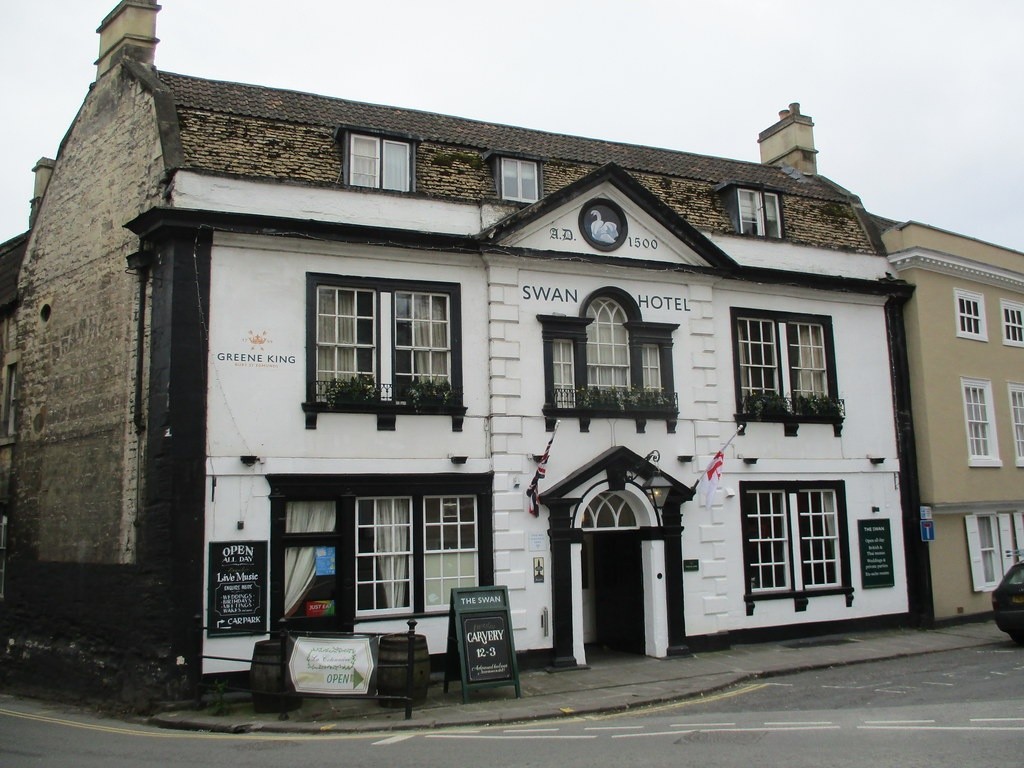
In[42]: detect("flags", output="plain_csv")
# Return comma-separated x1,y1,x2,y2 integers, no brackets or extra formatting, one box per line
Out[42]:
527,441,553,518
703,450,725,511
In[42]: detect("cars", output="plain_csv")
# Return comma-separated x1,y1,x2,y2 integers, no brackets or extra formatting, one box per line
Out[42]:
992,559,1024,645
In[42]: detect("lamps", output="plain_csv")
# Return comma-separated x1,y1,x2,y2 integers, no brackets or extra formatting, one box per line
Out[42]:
743,457,759,465
240,455,260,467
677,456,694,462
532,454,550,464
867,454,886,464
451,456,468,465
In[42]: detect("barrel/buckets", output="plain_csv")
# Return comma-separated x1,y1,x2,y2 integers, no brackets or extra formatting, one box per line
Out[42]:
378,632,430,707
249,640,303,712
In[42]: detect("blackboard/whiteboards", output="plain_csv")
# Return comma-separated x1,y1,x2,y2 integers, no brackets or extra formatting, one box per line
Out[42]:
207,540,269,639
857,518,895,590
443,585,520,688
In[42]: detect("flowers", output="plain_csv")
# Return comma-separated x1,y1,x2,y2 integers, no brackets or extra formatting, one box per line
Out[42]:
324,374,380,408
405,377,465,414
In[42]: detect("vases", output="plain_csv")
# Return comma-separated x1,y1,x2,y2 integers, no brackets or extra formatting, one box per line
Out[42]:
419,393,437,400
336,391,368,405
